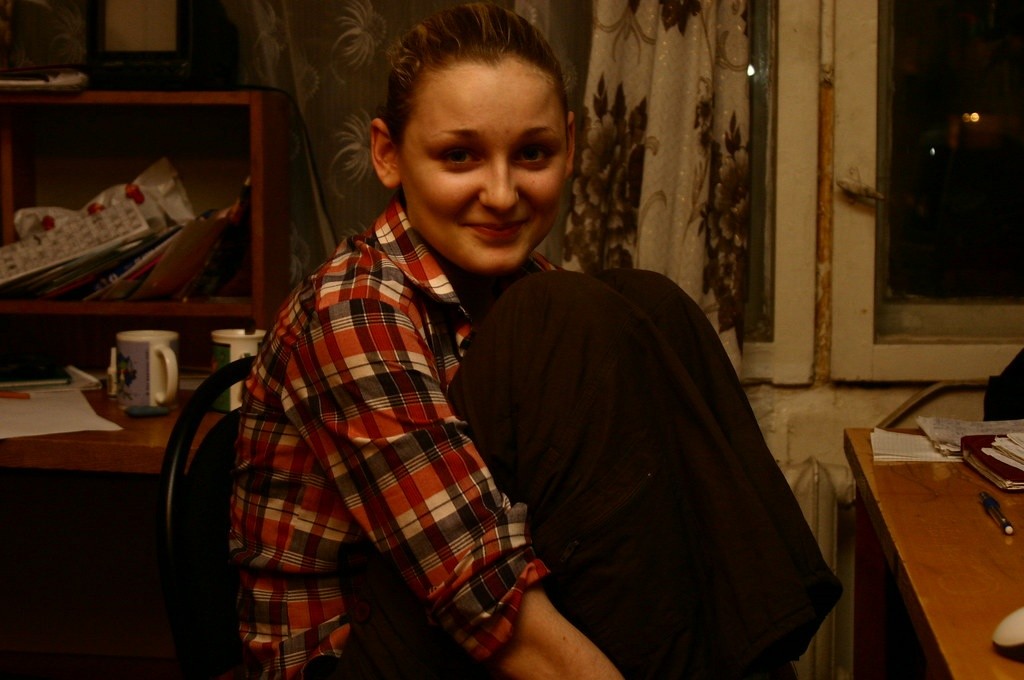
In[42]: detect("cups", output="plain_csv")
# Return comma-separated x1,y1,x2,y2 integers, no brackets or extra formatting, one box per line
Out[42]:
116,330,180,410
210,330,267,410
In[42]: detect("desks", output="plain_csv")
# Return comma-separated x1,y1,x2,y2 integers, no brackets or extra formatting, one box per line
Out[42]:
844,428,1024,680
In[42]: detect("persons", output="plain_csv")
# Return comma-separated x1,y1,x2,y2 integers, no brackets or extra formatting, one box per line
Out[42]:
222,0,840,680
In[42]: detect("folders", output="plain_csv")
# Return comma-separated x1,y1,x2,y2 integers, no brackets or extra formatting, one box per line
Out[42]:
962,434,1024,492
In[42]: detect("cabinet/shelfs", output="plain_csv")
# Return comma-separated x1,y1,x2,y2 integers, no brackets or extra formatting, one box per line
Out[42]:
0,81,292,680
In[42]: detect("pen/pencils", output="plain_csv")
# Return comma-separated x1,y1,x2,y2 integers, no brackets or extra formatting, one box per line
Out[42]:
979,491,1013,534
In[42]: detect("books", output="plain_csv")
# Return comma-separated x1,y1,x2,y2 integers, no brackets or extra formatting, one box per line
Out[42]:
871,416,1024,490
0,364,103,393
0,157,257,302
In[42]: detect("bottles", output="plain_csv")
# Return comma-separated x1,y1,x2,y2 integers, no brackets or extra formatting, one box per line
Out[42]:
107,348,116,396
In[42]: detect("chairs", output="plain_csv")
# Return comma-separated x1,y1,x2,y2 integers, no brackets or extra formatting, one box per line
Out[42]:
160,357,250,680
